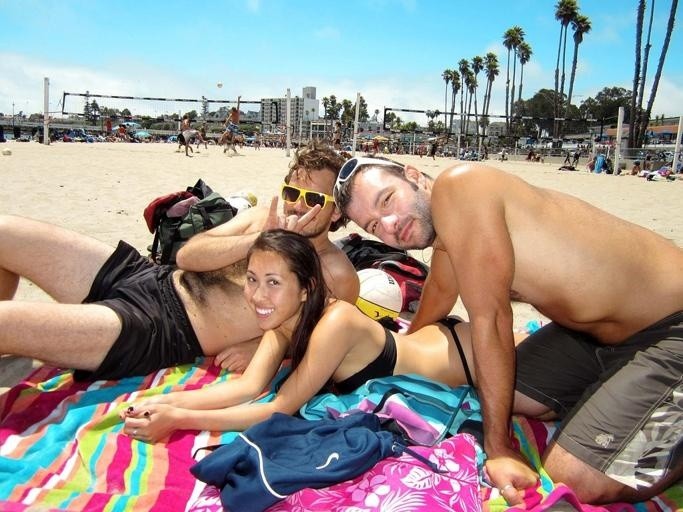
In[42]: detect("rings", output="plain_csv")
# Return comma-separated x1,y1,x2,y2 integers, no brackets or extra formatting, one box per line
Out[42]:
133,428,138,436
501,485,510,496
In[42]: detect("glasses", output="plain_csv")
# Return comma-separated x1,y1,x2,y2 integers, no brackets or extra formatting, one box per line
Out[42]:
280,183,339,213
333,153,409,212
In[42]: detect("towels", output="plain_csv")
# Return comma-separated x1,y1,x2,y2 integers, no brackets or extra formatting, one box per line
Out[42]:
0,317,680,510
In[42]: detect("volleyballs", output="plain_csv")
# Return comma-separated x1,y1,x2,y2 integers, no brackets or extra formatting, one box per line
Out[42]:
355,268,402,321
217,82,222,87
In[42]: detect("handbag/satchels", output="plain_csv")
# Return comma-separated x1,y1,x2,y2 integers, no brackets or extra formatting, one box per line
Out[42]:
151,176,239,267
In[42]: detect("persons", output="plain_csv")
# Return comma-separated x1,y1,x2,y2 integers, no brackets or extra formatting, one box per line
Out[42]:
332,122,509,162
332,155,683,505
119,227,528,449
2,116,287,158
525,145,640,175
221,95,242,153
0,137,358,382
